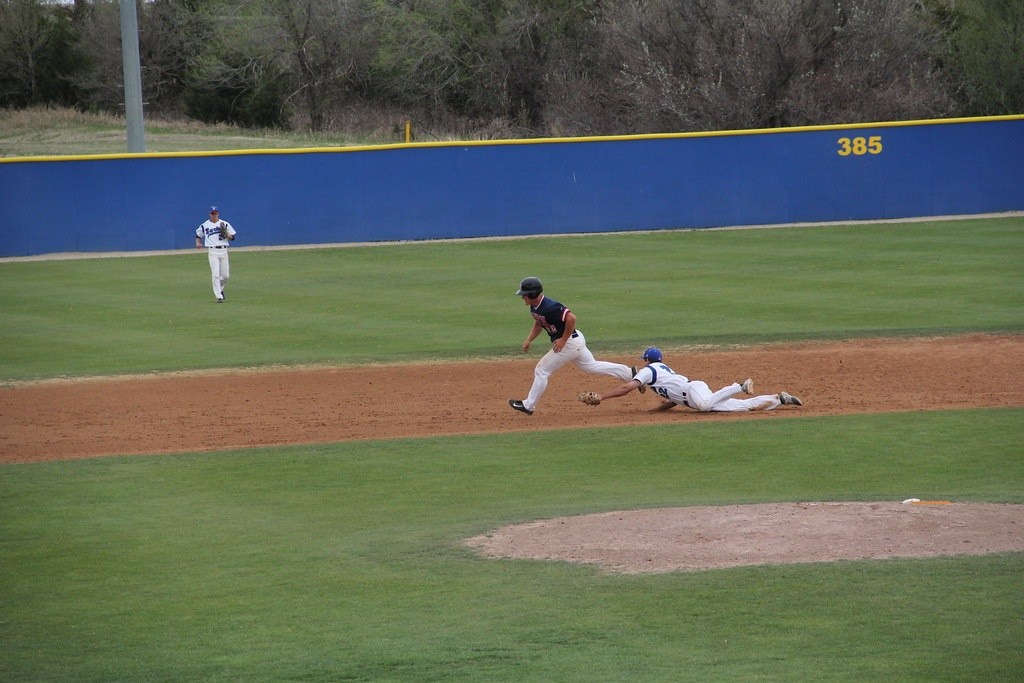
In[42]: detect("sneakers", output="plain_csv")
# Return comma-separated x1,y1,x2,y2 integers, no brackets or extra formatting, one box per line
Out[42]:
742,378,754,394
508,399,536,414
632,364,645,393
779,391,803,405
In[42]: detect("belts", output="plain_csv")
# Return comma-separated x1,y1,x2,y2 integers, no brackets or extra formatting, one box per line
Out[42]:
210,246,226,249
683,380,691,408
573,332,579,338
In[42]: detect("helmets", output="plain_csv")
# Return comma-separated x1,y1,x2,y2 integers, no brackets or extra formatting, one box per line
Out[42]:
516,277,542,299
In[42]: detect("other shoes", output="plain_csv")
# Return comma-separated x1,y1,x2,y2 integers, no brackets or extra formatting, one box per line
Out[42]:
220,291,225,298
217,298,223,302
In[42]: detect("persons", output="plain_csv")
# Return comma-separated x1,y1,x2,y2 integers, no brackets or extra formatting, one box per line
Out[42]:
509,277,646,414
580,347,803,412
197,206,236,302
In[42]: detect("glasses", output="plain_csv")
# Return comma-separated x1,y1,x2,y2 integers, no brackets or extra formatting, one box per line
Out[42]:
210,212,218,215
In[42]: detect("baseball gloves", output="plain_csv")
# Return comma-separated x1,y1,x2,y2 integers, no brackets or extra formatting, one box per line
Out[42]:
220,224,228,238
577,391,601,406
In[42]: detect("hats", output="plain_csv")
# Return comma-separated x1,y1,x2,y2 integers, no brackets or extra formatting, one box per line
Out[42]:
637,348,662,361
208,206,218,214
